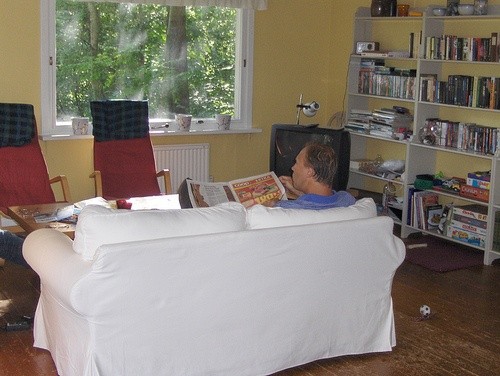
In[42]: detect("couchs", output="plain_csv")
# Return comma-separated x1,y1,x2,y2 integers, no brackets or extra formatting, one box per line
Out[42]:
22,196,407,376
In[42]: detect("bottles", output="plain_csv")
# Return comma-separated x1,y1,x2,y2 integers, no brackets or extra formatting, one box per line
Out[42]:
382,182,396,207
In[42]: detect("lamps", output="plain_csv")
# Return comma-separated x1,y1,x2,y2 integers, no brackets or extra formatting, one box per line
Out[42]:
294,93,320,126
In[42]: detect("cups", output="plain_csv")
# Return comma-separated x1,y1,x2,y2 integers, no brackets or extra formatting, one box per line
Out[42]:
216,114,231,131
474,0,488,16
175,113,192,133
371,0,410,17
72,117,89,136
447,0,460,16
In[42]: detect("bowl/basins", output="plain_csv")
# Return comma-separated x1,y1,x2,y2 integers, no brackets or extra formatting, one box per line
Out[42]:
433,8,446,15
457,4,474,16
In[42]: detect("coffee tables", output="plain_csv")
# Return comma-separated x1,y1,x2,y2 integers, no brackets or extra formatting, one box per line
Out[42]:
7,193,183,240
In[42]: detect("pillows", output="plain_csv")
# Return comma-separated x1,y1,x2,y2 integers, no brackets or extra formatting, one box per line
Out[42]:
72,201,247,261
245,197,378,230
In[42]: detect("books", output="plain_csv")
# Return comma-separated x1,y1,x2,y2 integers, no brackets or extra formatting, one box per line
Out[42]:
419,74,500,110
407,188,488,248
422,32,500,63
422,117,500,155
358,58,417,100
408,30,422,58
344,108,414,139
432,171,490,203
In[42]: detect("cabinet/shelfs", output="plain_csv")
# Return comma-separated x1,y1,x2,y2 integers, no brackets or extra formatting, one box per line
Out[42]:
339,0,500,267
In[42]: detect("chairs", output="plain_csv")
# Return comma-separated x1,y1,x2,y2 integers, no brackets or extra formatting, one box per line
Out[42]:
89,98,173,201
0,102,72,266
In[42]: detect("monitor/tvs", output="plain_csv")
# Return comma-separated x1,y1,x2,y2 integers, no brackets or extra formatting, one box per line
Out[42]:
270,124,351,192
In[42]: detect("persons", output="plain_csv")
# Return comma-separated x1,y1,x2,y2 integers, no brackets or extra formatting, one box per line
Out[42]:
179,143,356,210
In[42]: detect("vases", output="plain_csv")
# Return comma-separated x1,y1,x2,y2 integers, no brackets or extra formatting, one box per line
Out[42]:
446,0,460,16
473,0,489,15
370,0,398,17
175,114,192,133
70,117,89,135
215,114,232,131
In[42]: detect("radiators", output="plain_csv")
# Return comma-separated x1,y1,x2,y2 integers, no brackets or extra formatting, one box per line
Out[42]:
152,143,210,194
